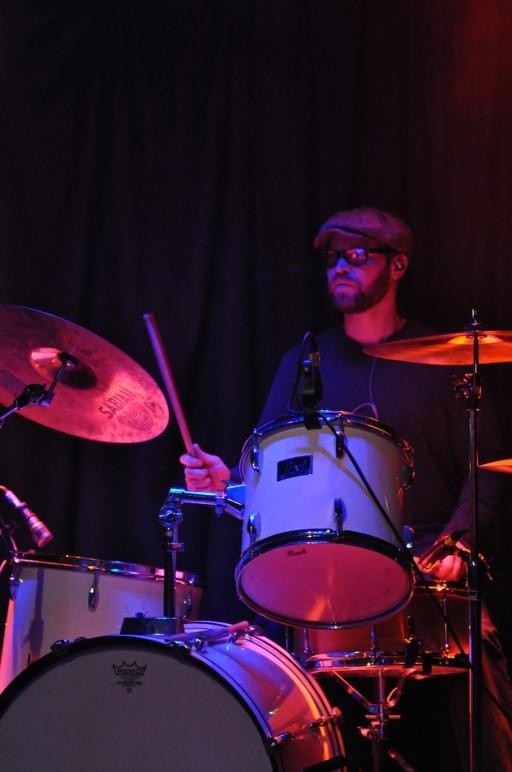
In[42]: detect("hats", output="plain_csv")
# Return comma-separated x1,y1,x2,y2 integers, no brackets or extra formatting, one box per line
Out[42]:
311,206,417,257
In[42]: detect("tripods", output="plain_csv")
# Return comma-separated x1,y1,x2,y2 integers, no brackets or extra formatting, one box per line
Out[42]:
328,671,414,772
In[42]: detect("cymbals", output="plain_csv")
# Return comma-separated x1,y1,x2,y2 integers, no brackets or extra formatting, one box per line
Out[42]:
1,302,170,445
410,577,477,603
364,329,510,367
310,647,472,682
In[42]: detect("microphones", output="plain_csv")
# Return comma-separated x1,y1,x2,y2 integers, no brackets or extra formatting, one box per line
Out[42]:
0,484,53,549
418,529,461,572
308,337,323,401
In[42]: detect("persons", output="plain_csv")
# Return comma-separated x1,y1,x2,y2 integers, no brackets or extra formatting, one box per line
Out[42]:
179,206,480,648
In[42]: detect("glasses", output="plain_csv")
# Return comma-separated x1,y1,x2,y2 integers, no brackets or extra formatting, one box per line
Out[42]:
313,247,395,271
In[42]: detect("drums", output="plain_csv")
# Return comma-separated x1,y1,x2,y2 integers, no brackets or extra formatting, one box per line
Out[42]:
12,551,198,692
233,410,412,630
1,623,344,772
